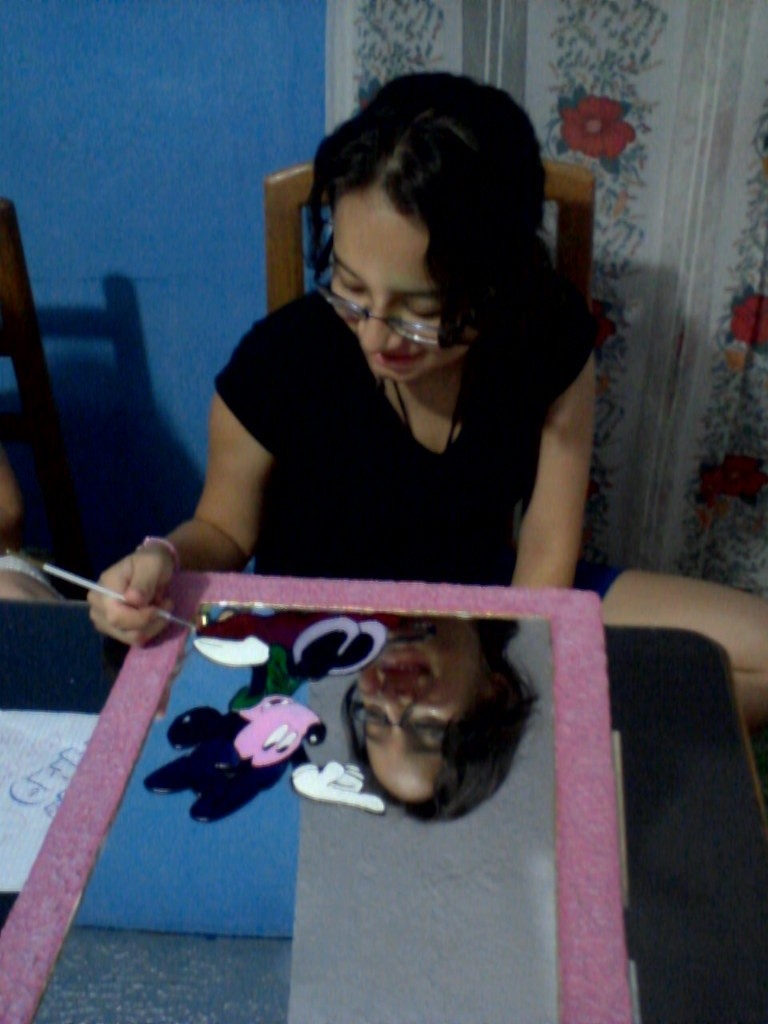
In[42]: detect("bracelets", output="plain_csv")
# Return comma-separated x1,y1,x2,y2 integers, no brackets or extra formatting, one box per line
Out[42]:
137,536,180,573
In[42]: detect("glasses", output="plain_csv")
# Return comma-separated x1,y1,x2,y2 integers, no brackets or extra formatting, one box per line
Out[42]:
350,703,445,753
312,235,443,345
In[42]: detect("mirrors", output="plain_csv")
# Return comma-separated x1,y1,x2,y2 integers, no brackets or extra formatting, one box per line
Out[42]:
0,572,627,1024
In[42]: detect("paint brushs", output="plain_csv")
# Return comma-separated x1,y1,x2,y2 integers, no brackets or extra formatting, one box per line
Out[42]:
43,563,197,632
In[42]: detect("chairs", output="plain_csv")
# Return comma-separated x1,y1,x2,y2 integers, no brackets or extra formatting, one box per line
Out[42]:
0,197,87,563
264,162,593,314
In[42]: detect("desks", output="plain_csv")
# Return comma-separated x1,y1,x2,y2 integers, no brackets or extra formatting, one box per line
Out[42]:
0,597,768,1024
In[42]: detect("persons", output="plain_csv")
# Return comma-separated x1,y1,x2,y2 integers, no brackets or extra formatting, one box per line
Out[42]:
87,72,768,734
341,615,526,824
1,445,67,603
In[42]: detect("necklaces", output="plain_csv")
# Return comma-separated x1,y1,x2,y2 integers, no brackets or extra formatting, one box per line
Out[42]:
392,379,460,450
